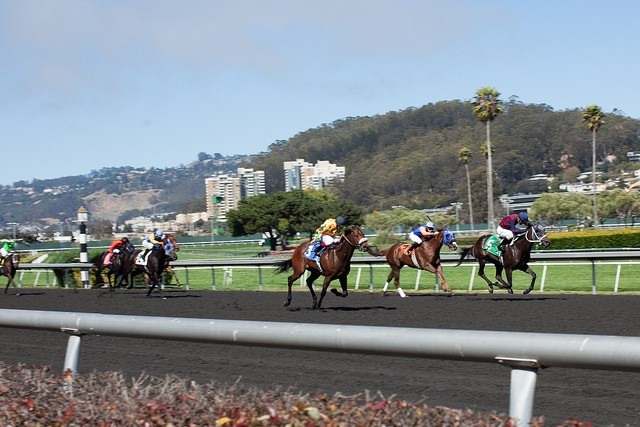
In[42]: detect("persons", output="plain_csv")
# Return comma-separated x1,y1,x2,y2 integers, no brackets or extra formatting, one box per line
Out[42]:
163,237,177,272
108,237,129,260
140,229,167,261
497,212,528,249
1,240,15,264
407,221,439,252
314,215,343,252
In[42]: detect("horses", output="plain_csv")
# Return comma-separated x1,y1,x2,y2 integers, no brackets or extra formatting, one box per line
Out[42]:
453,222,551,294
90,235,180,299
271,224,369,311
369,225,458,297
0,252,20,296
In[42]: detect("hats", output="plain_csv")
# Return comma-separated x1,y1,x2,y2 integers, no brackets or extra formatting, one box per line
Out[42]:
336,216,346,227
155,230,163,237
426,222,435,228
519,212,528,224
